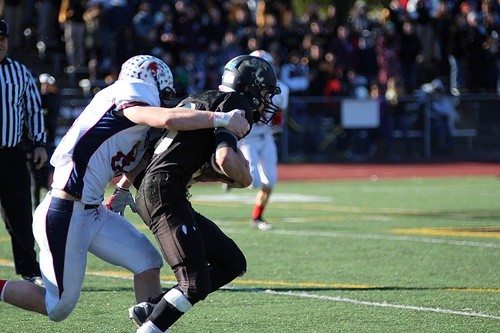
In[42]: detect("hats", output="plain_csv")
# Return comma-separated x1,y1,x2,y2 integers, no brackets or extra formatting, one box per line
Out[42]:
0,20,9,38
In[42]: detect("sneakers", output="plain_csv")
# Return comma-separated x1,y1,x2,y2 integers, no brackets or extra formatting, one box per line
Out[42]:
128,301,154,328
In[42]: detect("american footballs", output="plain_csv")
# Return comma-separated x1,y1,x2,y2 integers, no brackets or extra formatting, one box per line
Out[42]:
208,147,247,182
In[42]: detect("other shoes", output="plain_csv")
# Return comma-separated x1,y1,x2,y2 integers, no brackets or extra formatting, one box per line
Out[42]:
223,183,231,192
249,217,273,231
20,275,45,288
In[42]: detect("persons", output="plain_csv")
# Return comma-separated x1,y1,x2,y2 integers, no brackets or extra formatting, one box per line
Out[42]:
0,0,500,145
0,55,250,321
237,49,289,231
29,72,54,211
107,55,281,333
0,19,49,284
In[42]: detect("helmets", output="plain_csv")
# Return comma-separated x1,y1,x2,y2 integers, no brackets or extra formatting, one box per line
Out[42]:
222,55,281,125
118,55,177,107
248,50,274,66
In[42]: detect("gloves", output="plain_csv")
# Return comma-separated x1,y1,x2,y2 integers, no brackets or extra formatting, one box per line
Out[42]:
106,183,137,217
